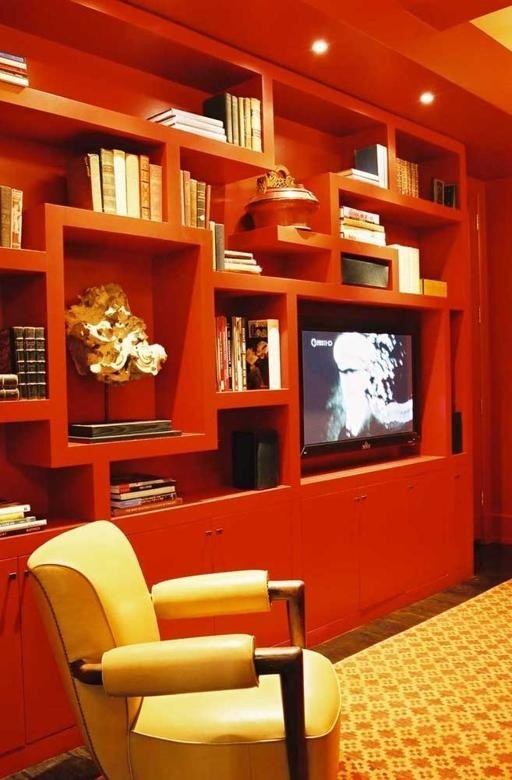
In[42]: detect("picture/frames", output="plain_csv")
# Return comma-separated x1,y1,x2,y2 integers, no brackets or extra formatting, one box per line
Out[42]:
434,179,445,206
444,184,457,208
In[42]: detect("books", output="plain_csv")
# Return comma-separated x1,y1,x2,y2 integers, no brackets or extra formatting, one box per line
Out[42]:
69,430,182,444
396,158,418,197
388,244,421,294
9,327,46,399
0,500,47,537
339,206,386,245
0,186,12,247
336,144,388,190
146,108,226,143
71,420,172,438
1,54,30,87
209,222,224,271
202,93,261,151
66,149,163,222
1,390,20,401
11,189,23,248
1,374,18,389
223,250,262,273
111,474,177,509
180,170,210,229
216,317,281,391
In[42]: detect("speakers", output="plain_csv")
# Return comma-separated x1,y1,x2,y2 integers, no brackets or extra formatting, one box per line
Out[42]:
232,428,280,491
452,411,463,454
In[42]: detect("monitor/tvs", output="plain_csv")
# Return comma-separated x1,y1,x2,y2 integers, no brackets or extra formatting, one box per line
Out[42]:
300,328,419,456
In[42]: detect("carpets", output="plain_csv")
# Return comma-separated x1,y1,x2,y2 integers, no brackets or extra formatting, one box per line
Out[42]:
335,577,512,780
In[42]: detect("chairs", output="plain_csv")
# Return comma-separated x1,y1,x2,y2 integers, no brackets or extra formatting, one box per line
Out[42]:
27,520,342,780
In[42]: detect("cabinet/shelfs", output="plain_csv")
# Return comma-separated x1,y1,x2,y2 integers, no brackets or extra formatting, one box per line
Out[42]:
0,0,476,779
